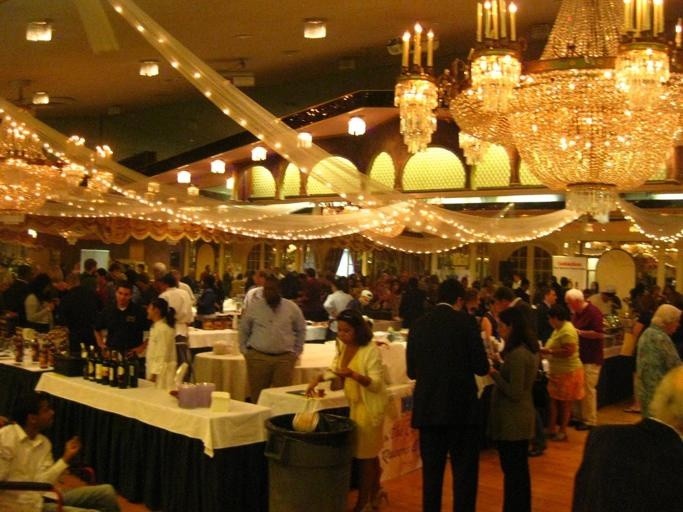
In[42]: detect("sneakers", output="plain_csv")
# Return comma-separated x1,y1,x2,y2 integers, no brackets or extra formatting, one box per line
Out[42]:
528,418,594,457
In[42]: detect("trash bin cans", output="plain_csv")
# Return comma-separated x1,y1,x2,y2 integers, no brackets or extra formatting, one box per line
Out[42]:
264,413,356,511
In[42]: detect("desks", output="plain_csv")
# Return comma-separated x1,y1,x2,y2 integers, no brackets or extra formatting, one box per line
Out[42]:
0,294,493,512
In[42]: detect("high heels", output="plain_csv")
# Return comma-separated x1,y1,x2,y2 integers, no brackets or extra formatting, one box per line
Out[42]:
358,488,389,511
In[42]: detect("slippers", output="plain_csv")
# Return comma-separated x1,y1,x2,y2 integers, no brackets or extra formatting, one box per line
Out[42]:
624,407,641,412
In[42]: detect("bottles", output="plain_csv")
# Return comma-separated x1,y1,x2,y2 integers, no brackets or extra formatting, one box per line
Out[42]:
12,327,138,388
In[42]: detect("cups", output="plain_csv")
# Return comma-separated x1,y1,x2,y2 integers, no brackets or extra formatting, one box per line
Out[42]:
211,392,230,411
177,379,215,408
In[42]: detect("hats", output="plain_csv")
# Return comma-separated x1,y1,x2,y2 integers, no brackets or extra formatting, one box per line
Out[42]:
362,290,373,300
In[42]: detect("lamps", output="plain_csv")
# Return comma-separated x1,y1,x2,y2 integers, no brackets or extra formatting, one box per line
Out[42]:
348,115,367,136
615,0,669,109
471,0,522,110
210,158,227,174
32,90,52,105
65,134,87,148
296,131,313,149
61,161,88,182
303,19,327,39
450,0,683,214
24,20,54,42
251,145,268,162
176,170,193,182
87,168,117,193
89,143,114,163
394,22,439,153
139,59,160,77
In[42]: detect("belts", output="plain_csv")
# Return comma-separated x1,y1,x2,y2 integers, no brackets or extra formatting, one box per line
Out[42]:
254,349,291,356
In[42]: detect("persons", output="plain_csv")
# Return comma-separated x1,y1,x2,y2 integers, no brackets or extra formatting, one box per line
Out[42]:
0,259,683,512
